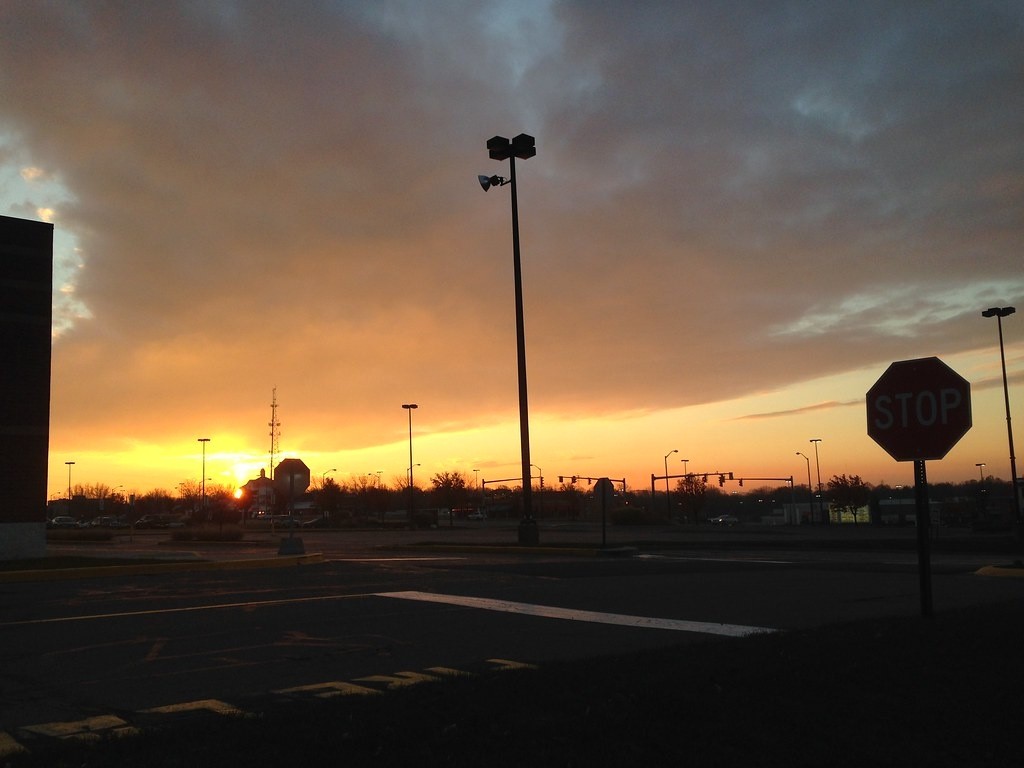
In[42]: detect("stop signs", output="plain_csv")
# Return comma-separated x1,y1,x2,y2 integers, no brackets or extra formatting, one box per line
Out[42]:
865,355,983,464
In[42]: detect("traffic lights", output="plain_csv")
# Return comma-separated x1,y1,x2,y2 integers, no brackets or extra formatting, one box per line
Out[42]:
589,479,592,484
739,479,743,487
559,476,563,483
702,474,707,482
719,477,722,487
572,478,577,484
541,476,543,489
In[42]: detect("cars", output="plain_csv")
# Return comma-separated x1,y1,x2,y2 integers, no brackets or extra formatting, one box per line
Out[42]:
303,517,338,528
467,512,488,521
136,517,186,528
710,514,739,525
272,514,299,528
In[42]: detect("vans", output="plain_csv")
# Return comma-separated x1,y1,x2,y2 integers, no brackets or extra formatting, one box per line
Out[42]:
50,516,76,529
92,517,111,527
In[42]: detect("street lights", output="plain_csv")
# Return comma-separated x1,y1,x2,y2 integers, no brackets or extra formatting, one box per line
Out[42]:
810,439,824,523
401,404,418,522
66,462,74,501
796,452,813,524
198,438,209,504
528,464,544,513
665,450,679,519
479,130,563,539
407,463,420,485
980,306,1021,525
682,459,688,479
